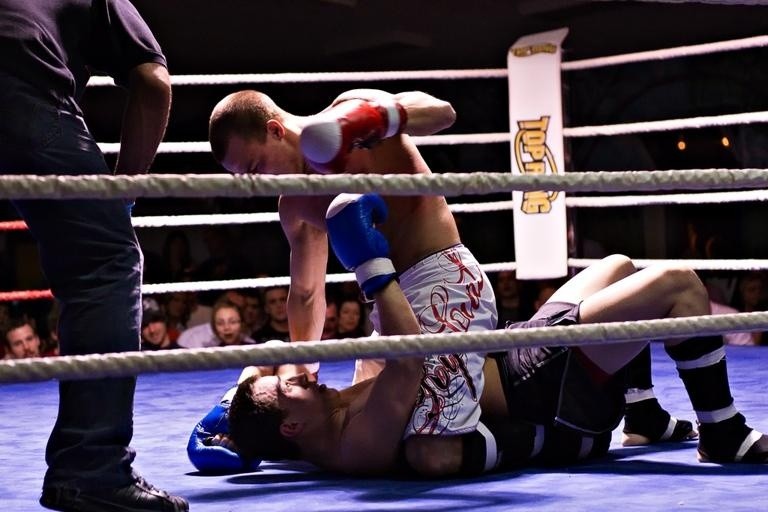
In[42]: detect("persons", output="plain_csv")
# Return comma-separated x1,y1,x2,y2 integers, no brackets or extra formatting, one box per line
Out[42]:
1,0,192,511
206,88,604,479
485,268,768,348
183,191,767,480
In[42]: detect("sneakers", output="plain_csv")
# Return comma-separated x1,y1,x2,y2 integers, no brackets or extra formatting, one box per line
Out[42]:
39,472,189,512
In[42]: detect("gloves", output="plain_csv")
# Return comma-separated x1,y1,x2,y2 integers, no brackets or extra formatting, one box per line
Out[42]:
324,191,400,299
185,384,263,475
297,90,407,174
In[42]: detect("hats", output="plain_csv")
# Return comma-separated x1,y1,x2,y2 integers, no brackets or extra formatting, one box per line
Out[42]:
141,298,165,332
